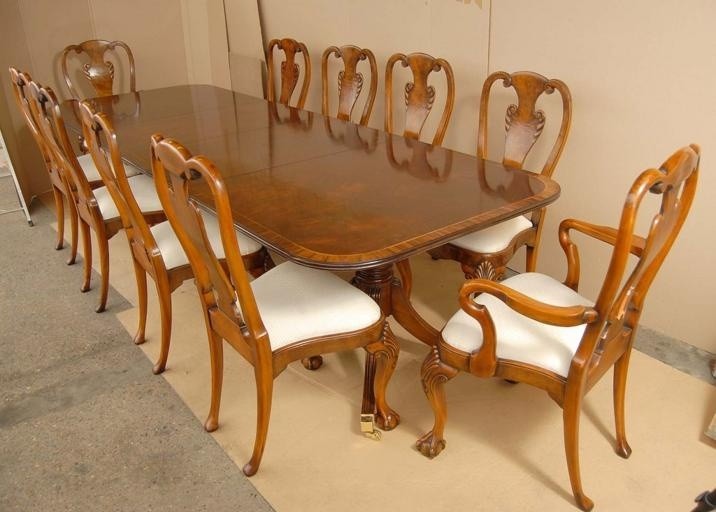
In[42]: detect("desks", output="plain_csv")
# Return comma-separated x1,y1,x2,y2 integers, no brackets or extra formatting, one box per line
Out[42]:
46,84,561,431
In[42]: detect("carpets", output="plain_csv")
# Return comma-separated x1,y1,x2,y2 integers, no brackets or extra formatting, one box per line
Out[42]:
47,196,716,509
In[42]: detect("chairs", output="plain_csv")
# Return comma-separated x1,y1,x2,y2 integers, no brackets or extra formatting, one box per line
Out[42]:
415,144,701,512
61,40,136,99
384,52,455,146
321,44,378,127
78,99,323,375
28,80,167,314
149,131,402,477
8,65,143,266
267,38,311,110
395,71,572,300
